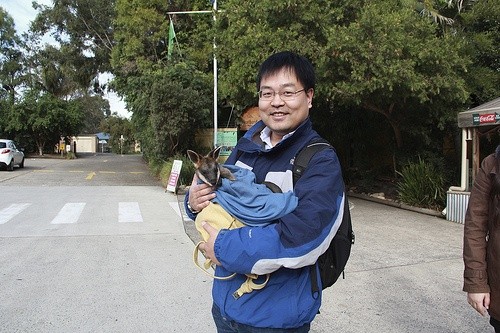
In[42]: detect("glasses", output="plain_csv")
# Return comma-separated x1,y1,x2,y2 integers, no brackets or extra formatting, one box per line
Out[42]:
256,88,306,101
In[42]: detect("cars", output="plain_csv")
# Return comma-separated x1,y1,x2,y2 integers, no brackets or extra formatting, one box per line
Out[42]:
0,139,25,171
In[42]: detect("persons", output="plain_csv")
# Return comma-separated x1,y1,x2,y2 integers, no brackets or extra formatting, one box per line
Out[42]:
464,145,500,333
183,50,345,333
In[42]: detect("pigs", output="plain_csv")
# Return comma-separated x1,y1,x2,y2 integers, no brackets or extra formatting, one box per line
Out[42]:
187,145,237,188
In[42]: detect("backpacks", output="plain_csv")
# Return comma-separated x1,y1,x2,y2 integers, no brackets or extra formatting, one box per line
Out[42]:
234,138,355,300
